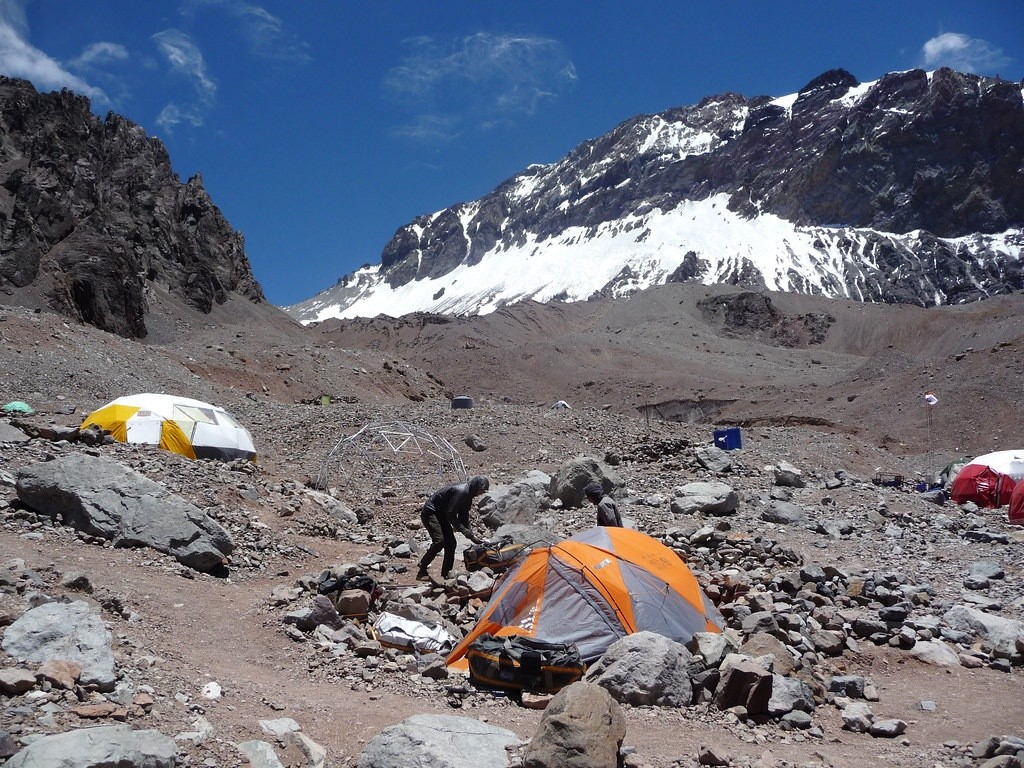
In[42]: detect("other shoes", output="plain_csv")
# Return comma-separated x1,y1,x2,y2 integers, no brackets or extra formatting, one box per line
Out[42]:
416,570,431,581
441,573,454,580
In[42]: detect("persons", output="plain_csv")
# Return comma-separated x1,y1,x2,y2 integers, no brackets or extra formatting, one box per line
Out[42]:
583,483,622,528
416,475,490,581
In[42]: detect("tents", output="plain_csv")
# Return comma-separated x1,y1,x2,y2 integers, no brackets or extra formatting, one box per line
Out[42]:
1008,480,1024,525
80,393,256,463
950,449,1024,509
443,526,724,678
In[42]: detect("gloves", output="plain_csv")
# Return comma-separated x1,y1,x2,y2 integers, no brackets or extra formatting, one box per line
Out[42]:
458,524,474,539
470,537,484,544
